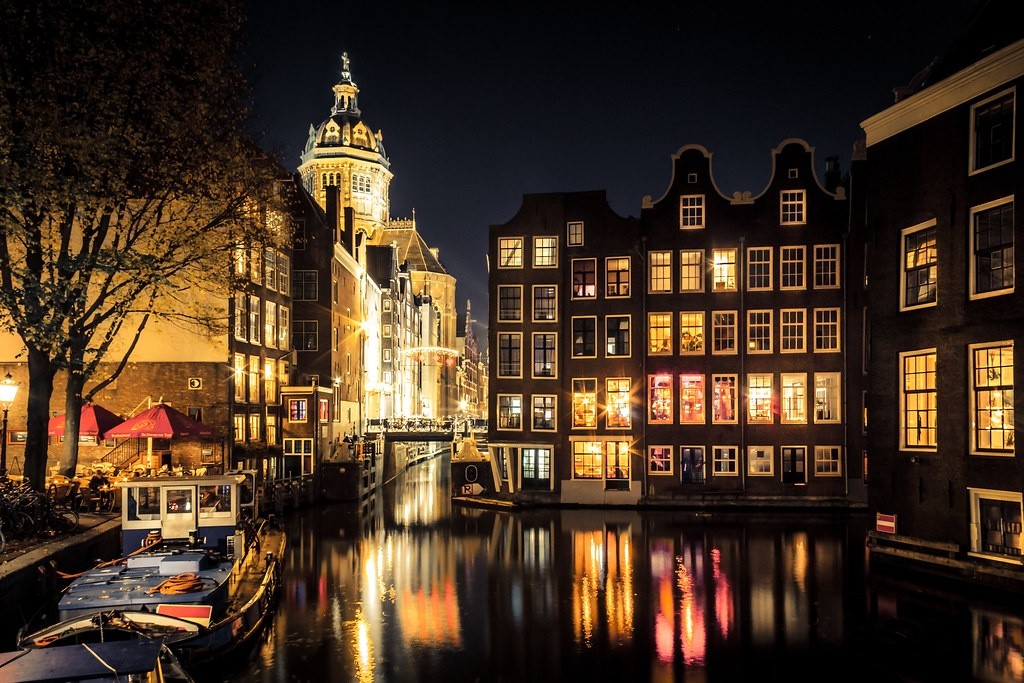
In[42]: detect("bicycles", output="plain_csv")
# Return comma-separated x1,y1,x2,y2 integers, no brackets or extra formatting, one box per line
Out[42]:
0,474,79,553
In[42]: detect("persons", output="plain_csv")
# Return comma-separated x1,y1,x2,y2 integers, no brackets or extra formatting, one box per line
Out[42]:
263,415,465,483
131,471,140,478
87,468,112,515
650,450,703,486
655,330,702,353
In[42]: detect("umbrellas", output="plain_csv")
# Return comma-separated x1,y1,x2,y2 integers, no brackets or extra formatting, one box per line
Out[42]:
103,402,217,442
48,401,128,440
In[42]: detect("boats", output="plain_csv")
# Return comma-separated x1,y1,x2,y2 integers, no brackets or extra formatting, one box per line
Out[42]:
1,612,198,683
15,470,288,667
